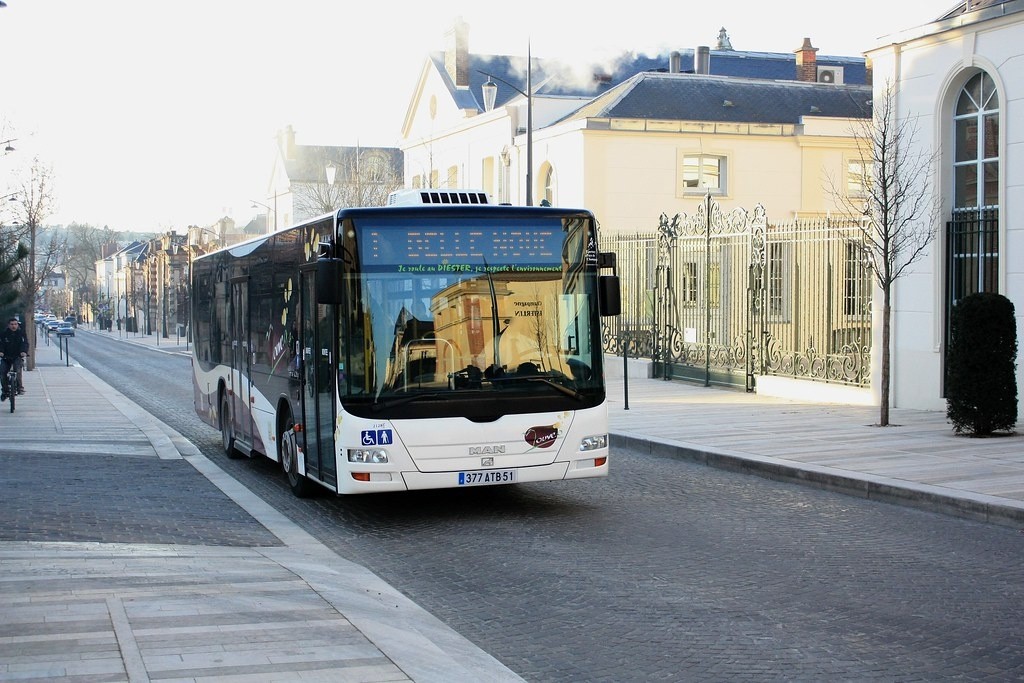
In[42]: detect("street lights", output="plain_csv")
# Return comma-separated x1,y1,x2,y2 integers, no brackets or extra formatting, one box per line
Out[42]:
115,269,128,339
250,200,276,230
202,228,225,249
684,179,710,387
323,157,361,207
173,242,192,343
151,253,167,338
123,266,135,336
99,275,110,320
136,260,151,335
473,69,533,206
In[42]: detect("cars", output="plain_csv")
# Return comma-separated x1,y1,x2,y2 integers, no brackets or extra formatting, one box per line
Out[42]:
56,322,75,338
46,321,62,333
64,317,77,328
34,310,57,329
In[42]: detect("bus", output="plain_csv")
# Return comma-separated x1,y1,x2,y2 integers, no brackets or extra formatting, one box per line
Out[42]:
191,189,621,497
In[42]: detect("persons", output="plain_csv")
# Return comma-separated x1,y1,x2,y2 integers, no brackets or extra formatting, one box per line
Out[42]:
288,318,299,378
116,316,126,330
397,301,436,352
303,319,314,374
0,318,29,401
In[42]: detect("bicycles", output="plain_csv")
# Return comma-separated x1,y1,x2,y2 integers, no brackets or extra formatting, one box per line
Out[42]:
0,354,30,413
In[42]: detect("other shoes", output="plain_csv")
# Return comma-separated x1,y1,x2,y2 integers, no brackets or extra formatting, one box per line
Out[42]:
17,386,24,394
1,392,7,401
